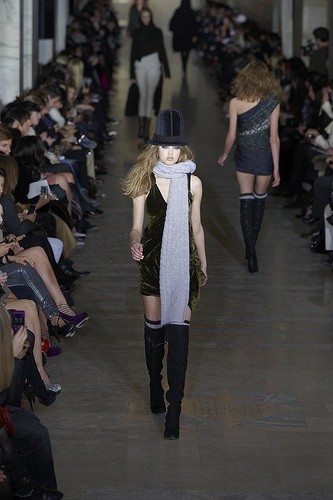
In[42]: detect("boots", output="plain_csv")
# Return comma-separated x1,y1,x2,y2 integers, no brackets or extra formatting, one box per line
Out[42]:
239,191,268,273
138,117,151,141
163,319,190,439
144,314,166,414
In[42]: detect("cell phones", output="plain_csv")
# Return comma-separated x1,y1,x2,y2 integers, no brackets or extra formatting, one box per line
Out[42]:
27,205,37,215
40,186,48,199
12,311,25,334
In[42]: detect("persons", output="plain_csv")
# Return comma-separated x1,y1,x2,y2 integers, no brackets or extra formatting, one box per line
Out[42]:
217,60,281,274
168,0,198,82
123,7,171,139
127,0,149,44
195,0,333,263
1,0,123,499
121,109,208,441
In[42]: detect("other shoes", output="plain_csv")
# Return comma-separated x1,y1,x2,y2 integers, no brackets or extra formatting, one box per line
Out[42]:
44,490,63,500
24,125,118,413
268,175,333,264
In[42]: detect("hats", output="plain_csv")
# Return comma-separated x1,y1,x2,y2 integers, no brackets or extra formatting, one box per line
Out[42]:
146,110,192,145
